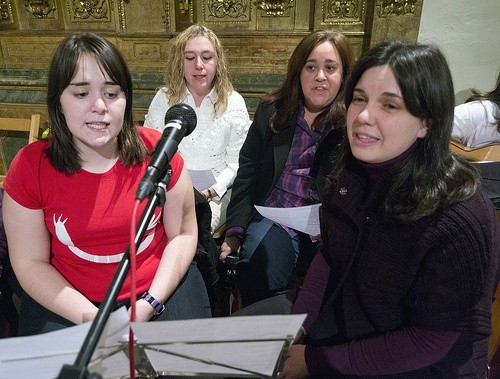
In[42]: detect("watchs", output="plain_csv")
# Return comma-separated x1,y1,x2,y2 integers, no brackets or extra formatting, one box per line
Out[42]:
207,188,211,202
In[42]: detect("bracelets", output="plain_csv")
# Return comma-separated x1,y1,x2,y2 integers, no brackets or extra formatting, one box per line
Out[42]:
141,291,165,316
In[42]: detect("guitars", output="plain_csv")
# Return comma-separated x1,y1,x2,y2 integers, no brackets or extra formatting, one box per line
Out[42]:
448,138,500,163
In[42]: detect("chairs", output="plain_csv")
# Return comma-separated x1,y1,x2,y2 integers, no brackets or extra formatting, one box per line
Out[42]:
0,114,41,182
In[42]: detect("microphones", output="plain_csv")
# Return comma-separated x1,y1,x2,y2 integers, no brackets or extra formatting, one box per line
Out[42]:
134,103,197,202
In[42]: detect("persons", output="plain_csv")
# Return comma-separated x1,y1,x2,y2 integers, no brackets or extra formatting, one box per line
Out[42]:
228,39,500,379
219,29,358,306
2,33,213,338
142,24,250,234
